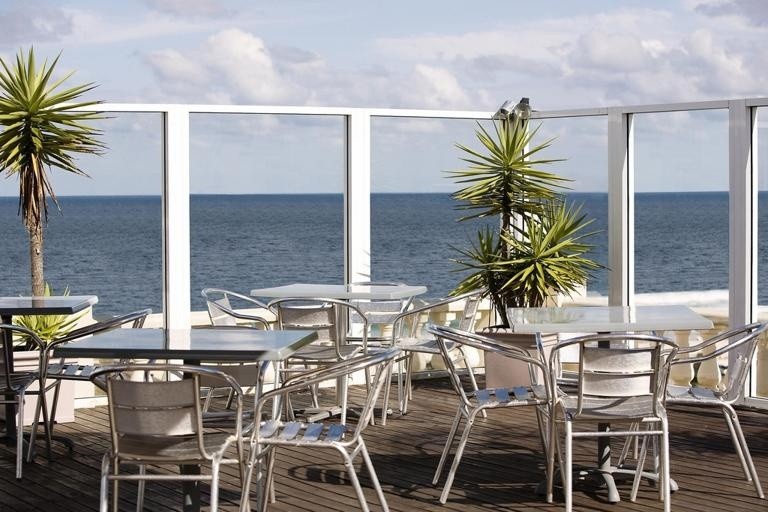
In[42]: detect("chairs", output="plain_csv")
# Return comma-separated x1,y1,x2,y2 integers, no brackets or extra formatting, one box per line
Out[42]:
349,281,413,409
544,333,679,512
426,322,568,505
1,324,43,480
232,346,403,512
617,318,766,511
200,286,282,414
380,287,489,427
86,362,246,512
26,308,153,463
268,294,376,438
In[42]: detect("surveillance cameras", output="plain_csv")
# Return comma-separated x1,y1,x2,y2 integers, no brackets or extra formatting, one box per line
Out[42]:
499,100,516,115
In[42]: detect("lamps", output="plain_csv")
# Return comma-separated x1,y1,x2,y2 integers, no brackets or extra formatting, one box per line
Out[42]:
514,96,534,122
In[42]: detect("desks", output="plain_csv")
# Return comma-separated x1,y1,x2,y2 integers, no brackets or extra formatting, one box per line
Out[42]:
250,282,428,425
52,328,319,510
1,295,98,459
508,304,716,503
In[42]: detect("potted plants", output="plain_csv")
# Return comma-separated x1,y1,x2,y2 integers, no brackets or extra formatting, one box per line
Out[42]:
443,110,617,398
0,42,119,429
